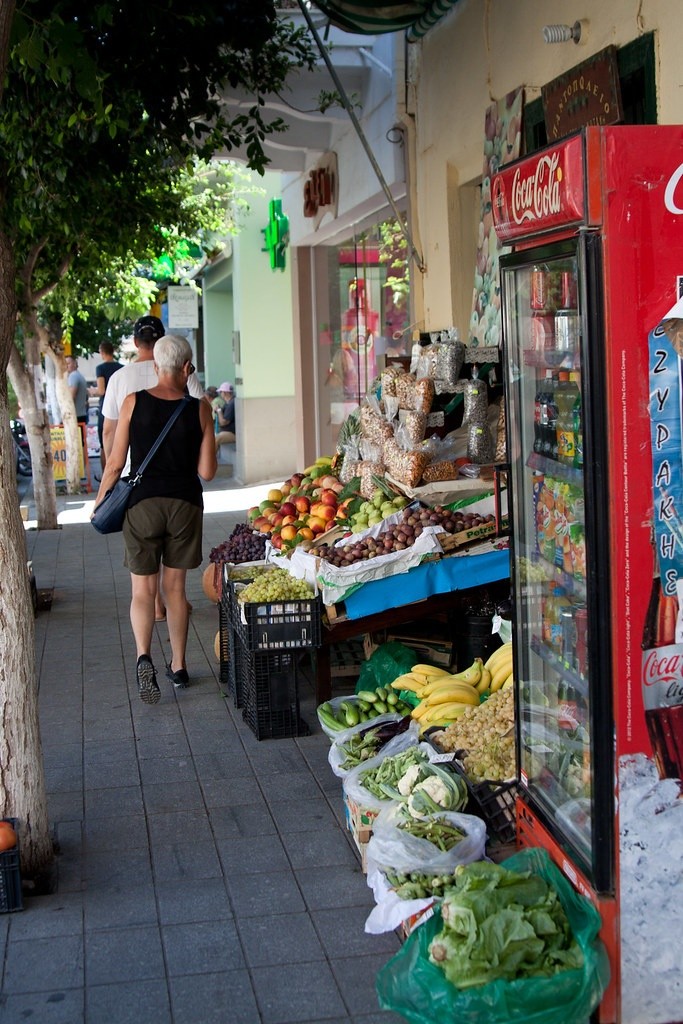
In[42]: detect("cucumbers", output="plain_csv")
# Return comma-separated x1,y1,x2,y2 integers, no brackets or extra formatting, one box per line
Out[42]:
318,683,411,731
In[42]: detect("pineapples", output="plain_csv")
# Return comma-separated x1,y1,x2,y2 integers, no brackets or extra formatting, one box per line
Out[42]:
333,455,345,477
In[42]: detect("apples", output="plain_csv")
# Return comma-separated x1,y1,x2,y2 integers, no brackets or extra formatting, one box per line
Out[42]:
248,467,323,522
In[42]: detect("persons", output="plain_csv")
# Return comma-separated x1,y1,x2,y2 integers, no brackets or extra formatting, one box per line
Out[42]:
56,355,89,482
204,382,236,453
102,315,212,621
89,334,218,705
86,341,125,482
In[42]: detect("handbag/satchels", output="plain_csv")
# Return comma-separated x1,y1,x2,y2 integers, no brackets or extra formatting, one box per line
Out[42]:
90,477,132,534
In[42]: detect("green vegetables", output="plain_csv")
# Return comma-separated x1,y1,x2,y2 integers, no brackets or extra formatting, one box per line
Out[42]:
385,862,584,990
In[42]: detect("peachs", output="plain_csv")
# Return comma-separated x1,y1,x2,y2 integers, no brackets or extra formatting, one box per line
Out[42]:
254,476,354,552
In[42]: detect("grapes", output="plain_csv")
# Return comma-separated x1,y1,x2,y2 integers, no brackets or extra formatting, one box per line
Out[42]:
227,564,314,602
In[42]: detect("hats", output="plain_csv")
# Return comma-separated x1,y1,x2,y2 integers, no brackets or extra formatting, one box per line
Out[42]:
134,316,165,337
216,382,234,392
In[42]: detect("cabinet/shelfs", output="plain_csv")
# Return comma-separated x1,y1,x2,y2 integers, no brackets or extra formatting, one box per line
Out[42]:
382,343,501,442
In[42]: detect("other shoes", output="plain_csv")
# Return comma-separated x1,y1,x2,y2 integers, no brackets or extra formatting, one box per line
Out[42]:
95,473,103,482
188,603,193,614
155,606,167,622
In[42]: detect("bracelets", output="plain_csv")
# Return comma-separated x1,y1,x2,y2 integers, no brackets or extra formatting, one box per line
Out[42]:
217,408,222,412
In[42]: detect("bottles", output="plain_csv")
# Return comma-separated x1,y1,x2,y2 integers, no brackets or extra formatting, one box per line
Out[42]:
642,535,683,786
528,366,590,768
342,279,378,396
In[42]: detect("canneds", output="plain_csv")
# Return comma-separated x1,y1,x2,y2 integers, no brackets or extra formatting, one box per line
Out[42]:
531,267,578,354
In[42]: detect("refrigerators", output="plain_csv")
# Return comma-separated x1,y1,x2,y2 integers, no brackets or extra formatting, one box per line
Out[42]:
488,122,683,1024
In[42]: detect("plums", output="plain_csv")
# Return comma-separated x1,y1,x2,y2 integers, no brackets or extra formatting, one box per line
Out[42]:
208,523,267,563
297,505,495,568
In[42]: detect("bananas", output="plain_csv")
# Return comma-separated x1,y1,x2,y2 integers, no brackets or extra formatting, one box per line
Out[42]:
303,457,331,474
391,642,512,738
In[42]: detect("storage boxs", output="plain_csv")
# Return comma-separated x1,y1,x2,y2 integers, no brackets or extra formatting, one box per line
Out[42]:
218,560,513,943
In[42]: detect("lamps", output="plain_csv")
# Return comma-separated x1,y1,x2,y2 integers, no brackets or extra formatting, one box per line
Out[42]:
541,18,589,48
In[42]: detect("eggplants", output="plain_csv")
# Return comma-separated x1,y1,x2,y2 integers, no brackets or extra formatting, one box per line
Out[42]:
351,489,407,533
335,715,412,767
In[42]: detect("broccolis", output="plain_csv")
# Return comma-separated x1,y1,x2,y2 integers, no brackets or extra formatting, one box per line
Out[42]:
397,761,434,800
406,763,467,819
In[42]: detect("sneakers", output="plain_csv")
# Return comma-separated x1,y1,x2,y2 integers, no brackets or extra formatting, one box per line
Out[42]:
136,654,161,705
165,660,189,688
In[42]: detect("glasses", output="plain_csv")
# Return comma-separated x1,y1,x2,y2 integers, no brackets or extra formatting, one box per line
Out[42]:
190,363,195,374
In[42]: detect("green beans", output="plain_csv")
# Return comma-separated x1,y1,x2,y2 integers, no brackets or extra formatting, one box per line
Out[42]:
360,746,466,851
